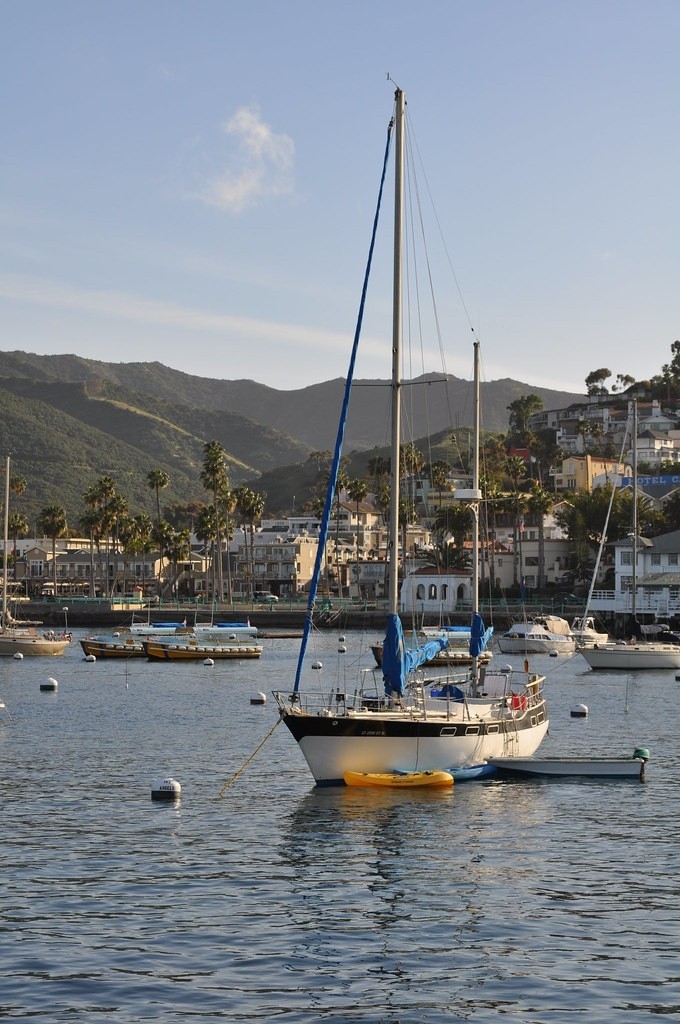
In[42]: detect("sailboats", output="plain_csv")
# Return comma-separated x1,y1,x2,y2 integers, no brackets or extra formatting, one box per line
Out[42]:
0,452,73,657
267,70,552,788
563,397,680,671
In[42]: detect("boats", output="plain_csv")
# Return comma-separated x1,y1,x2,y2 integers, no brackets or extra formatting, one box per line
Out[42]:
570,615,610,649
342,767,454,789
497,612,577,654
483,746,651,781
80,595,263,663
367,640,495,667
402,603,471,644
395,762,498,780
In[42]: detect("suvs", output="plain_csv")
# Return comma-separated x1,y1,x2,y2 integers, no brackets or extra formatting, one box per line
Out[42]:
253,591,278,603
